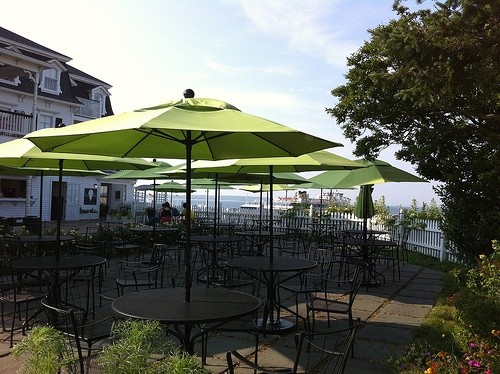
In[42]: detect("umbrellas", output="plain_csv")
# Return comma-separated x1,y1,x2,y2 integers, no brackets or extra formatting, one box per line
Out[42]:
0,88,432,354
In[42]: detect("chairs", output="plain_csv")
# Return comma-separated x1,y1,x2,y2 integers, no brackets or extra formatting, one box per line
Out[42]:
0,216,400,374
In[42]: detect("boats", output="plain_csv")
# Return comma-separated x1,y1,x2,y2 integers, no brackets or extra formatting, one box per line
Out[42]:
240,200,260,211
264,189,352,210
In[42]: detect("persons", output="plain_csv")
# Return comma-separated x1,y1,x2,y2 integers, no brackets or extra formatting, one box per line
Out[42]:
181,203,194,218
161,201,179,217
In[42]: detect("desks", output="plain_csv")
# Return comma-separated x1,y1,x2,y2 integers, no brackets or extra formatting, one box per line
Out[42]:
2,235,75,287
129,226,179,265
341,230,389,265
225,256,319,335
6,254,107,330
111,285,262,359
334,238,396,287
183,235,246,284
233,230,286,256
